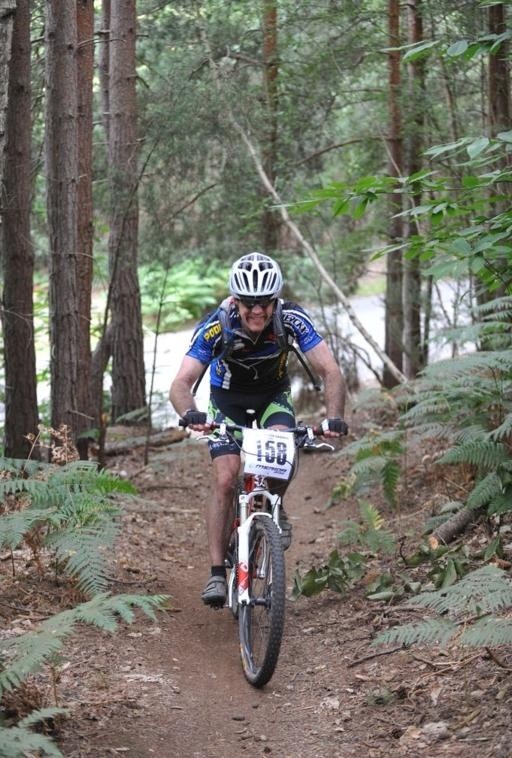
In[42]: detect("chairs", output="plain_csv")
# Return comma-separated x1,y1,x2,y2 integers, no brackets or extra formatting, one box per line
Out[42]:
202,573,228,606
268,508,292,550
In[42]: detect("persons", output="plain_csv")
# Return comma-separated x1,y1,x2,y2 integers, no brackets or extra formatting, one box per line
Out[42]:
169,251,346,609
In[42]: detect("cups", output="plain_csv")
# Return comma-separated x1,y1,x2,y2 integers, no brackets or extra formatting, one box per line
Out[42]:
234,298,276,308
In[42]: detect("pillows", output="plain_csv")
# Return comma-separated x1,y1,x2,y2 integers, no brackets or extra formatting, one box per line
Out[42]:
229,252,283,311
